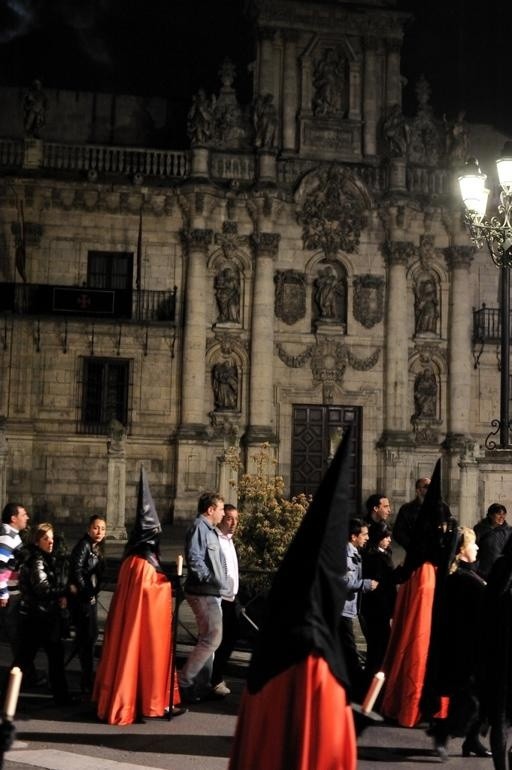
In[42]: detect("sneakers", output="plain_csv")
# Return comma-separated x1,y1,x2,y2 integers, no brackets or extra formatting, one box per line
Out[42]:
214,681,233,697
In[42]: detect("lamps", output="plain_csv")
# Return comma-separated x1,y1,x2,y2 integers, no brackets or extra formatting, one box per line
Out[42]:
455,134,511,270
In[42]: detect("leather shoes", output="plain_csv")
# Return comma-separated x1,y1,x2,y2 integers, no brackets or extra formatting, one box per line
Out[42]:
155,703,189,721
460,741,495,759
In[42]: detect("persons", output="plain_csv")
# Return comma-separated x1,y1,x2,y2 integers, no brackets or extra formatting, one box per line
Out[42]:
312,49,342,119
418,282,438,332
382,100,472,160
186,78,281,150
215,267,239,321
414,368,437,418
213,360,238,410
314,266,338,317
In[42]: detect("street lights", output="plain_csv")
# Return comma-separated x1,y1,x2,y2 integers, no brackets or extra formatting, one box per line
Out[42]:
456,141,512,451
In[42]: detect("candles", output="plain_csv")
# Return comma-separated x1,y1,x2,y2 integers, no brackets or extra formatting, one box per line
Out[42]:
4,665,24,722
176,553,184,578
359,670,385,716
